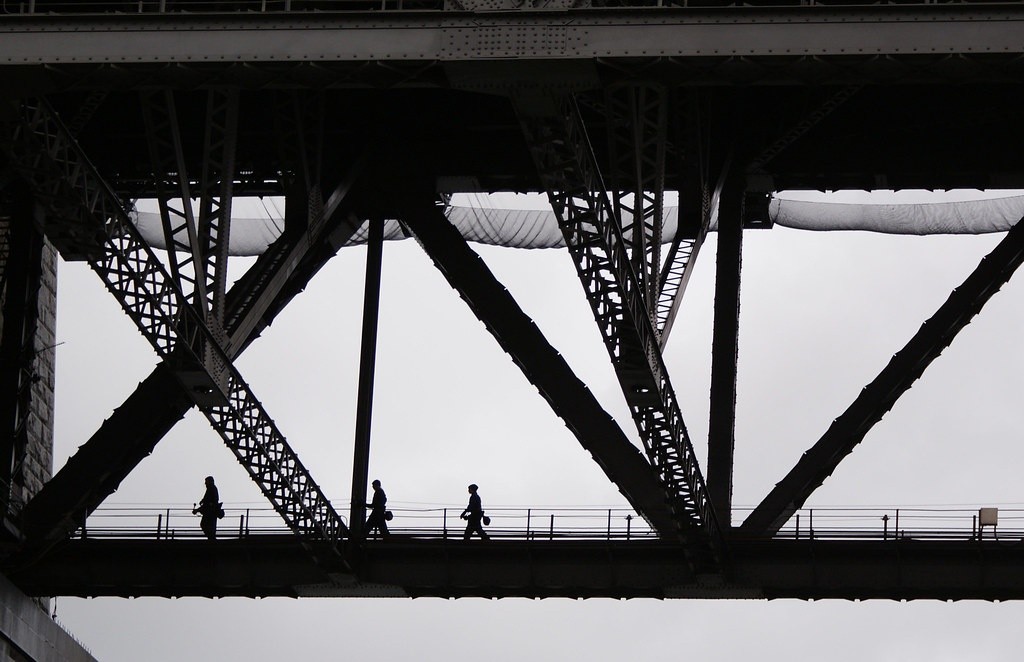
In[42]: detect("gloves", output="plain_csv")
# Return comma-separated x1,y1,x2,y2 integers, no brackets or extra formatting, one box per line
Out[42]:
460,510,466,519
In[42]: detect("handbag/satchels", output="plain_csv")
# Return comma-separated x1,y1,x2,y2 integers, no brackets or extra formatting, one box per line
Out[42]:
217,502,225,519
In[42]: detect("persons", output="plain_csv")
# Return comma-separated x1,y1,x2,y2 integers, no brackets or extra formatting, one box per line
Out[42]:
460,484,490,540
366,480,390,533
193,476,219,539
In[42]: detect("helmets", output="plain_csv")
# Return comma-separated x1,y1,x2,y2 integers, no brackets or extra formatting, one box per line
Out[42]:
483,516,490,526
384,510,393,522
